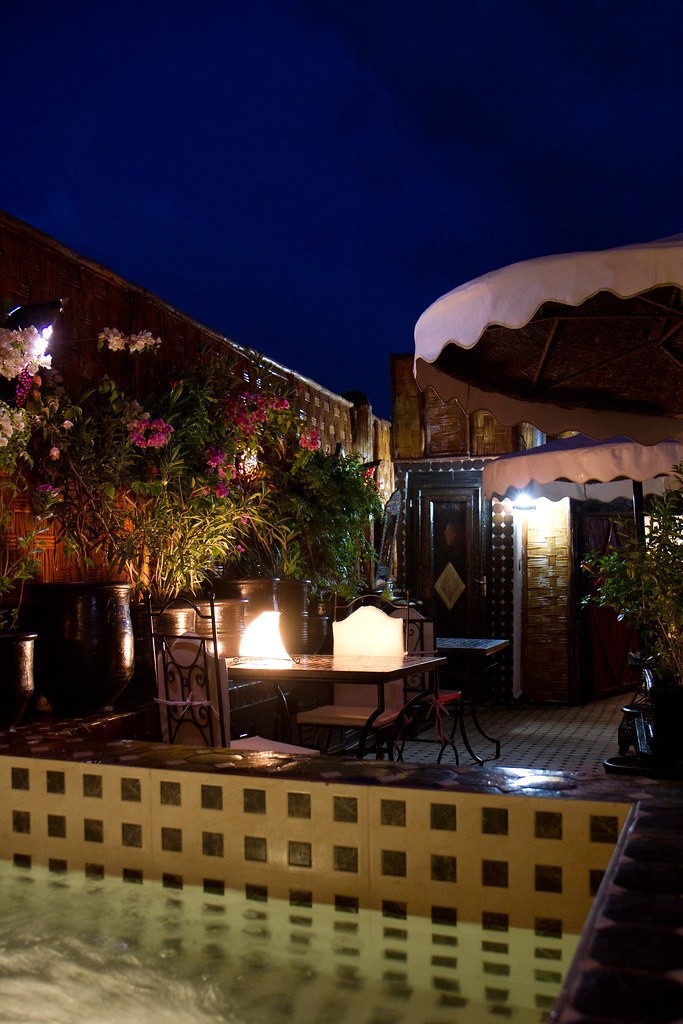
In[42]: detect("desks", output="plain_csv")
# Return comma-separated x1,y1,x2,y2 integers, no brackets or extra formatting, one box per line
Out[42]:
436,636,510,767
225,654,448,762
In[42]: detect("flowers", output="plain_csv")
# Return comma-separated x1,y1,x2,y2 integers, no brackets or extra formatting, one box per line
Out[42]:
0,327,321,637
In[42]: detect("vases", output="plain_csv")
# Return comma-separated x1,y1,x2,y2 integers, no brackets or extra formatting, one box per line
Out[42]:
28,581,135,720
131,598,250,700
0,632,37,730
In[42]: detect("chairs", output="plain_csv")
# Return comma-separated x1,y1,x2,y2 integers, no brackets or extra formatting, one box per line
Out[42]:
144,590,319,755
296,590,411,762
380,596,462,765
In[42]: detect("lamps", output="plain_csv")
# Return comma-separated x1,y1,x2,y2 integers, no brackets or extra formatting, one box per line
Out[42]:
0,298,66,406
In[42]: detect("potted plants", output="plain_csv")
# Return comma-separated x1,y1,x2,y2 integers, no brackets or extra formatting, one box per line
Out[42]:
215,447,387,656
580,459,683,776
